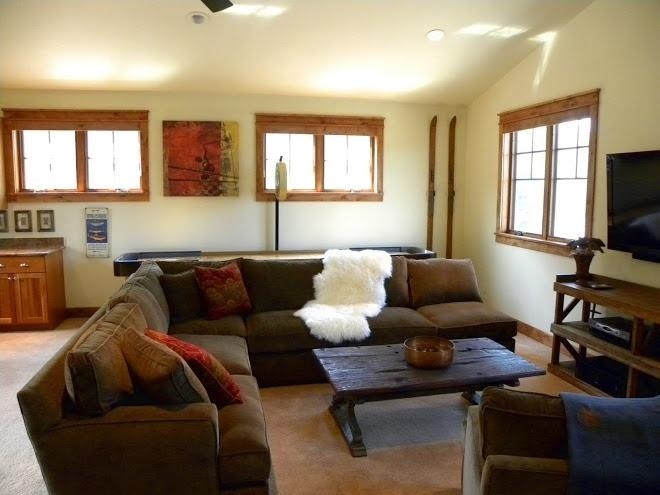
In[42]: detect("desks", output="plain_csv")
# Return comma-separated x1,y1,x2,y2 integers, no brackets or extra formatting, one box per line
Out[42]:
114,247,437,276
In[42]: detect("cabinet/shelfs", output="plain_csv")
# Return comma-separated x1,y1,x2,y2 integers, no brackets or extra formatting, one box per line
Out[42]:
0,239,65,331
548,275,660,400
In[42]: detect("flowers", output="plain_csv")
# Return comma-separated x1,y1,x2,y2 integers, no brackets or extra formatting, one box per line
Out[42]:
568,238,605,254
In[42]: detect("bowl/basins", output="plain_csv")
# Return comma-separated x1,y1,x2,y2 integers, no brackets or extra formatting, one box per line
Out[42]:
403,336,455,369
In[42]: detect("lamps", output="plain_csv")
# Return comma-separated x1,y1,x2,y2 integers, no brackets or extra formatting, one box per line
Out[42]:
275,156,287,250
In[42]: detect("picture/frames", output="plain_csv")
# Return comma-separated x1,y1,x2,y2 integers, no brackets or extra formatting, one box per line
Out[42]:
0,210,8,231
14,211,32,232
37,210,55,232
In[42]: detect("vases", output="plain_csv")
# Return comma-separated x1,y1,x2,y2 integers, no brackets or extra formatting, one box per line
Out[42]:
574,254,595,274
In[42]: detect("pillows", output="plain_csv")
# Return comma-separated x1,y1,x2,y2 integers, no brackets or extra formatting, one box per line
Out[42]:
121,330,212,404
160,271,204,323
146,331,243,409
195,262,252,320
407,258,482,307
68,302,148,412
109,260,172,334
157,257,243,273
245,256,411,313
477,387,569,459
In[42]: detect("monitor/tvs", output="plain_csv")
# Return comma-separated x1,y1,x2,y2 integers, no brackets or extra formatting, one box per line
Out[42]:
606,151,660,263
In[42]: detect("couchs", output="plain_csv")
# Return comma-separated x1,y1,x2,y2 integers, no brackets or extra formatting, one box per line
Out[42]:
17,258,516,495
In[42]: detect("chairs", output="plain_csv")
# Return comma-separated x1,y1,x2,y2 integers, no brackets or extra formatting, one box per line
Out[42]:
463,387,659,495
